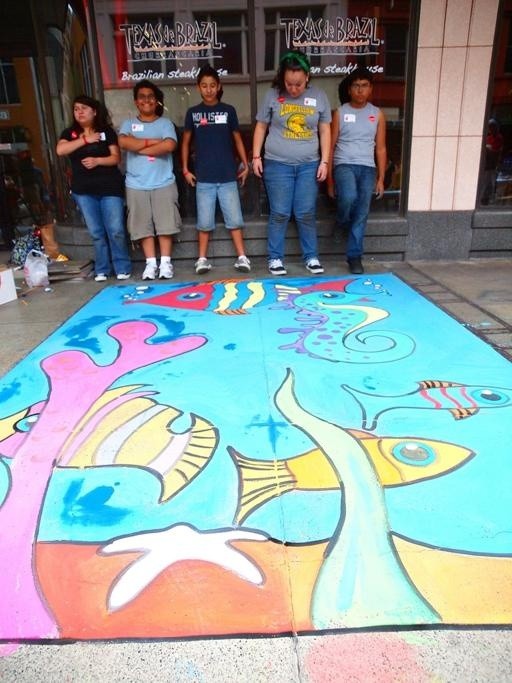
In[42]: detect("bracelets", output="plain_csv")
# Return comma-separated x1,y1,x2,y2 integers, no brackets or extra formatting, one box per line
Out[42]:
252,157,261,159
320,161,328,165
327,179,334,185
183,171,189,177
82,135,88,145
144,139,149,148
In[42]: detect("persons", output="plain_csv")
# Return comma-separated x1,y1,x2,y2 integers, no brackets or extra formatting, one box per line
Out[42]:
482,118,504,206
252,48,332,276
326,68,387,274
180,66,251,274
118,79,182,280
56,95,132,282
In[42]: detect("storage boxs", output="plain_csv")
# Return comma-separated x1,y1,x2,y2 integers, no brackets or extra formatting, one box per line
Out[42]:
0,267,18,307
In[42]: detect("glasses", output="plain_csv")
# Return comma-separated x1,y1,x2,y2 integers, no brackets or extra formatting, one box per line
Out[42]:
353,83,368,89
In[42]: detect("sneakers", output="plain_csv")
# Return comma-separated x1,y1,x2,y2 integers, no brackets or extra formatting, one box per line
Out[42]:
142,264,158,280
331,222,345,243
116,273,131,279
158,262,174,279
347,258,365,274
267,259,287,276
194,259,212,273
233,257,251,273
304,257,324,274
94,274,113,282
